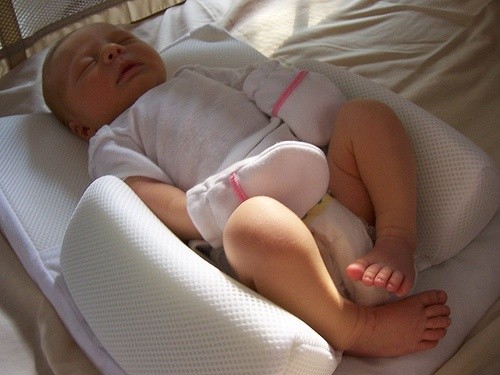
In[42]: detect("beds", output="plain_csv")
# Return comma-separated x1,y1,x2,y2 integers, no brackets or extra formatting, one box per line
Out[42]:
0,0,500,375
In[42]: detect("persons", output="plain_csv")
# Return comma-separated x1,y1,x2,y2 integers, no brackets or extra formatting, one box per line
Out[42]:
42,22,452,359
